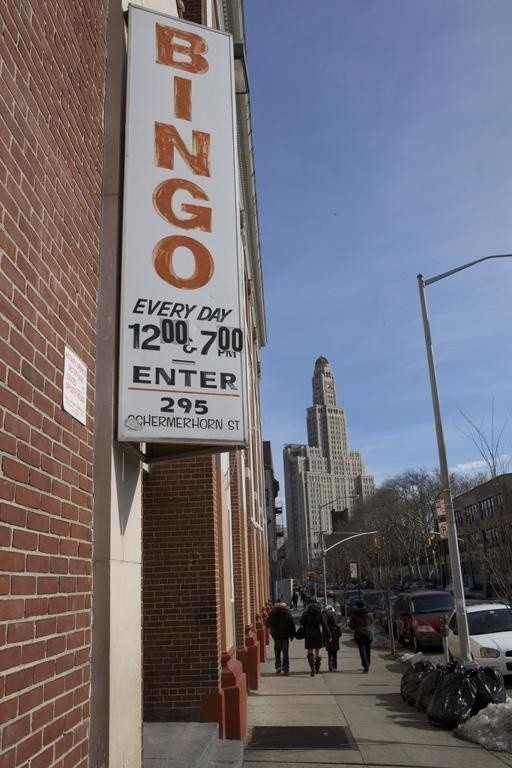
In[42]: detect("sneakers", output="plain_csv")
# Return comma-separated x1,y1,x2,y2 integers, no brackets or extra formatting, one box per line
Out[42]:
275,667,292,676
328,660,371,674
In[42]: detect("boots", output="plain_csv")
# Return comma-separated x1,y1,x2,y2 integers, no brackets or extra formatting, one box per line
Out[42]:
316,656,323,674
305,653,317,678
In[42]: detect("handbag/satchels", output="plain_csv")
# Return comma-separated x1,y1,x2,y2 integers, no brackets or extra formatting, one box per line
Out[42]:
295,626,305,640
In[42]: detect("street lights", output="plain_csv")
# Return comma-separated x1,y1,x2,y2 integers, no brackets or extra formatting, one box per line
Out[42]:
318,494,360,605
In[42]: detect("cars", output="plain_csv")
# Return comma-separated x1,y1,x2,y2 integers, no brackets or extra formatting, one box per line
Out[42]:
317,587,334,597
341,577,512,677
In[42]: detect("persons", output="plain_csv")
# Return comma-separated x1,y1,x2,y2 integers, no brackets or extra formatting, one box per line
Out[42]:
301,591,307,609
322,614,343,672
292,592,299,611
399,576,436,591
366,578,375,589
300,603,333,677
266,600,296,676
349,600,375,673
